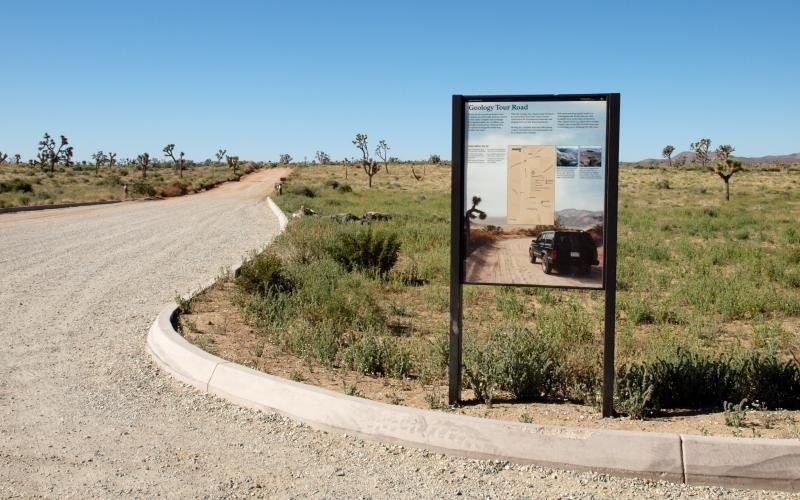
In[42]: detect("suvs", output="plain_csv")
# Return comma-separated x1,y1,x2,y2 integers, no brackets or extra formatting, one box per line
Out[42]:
530,228,600,277
